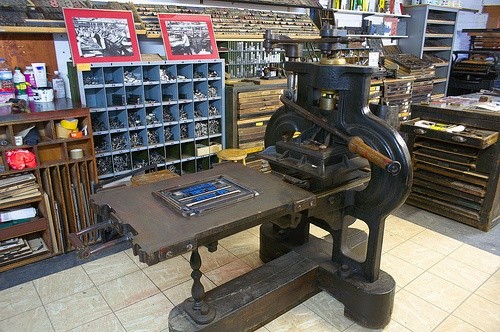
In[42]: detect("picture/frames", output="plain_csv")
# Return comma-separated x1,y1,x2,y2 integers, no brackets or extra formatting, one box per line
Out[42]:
157,12,220,60
61,7,142,65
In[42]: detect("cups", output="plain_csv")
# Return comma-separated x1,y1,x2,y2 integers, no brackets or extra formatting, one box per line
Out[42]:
71,149,83,159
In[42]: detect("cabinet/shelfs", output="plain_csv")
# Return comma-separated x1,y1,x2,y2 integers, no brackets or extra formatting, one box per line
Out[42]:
315,7,410,70
0,107,109,274
68,57,227,193
393,91,500,231
397,5,459,99
223,77,292,159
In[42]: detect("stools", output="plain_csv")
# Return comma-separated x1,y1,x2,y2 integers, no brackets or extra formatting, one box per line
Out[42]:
130,169,180,187
216,148,247,167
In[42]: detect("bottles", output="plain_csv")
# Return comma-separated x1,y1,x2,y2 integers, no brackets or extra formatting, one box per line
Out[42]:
0,58,13,88
25,66,36,100
13,67,28,101
52,71,65,98
